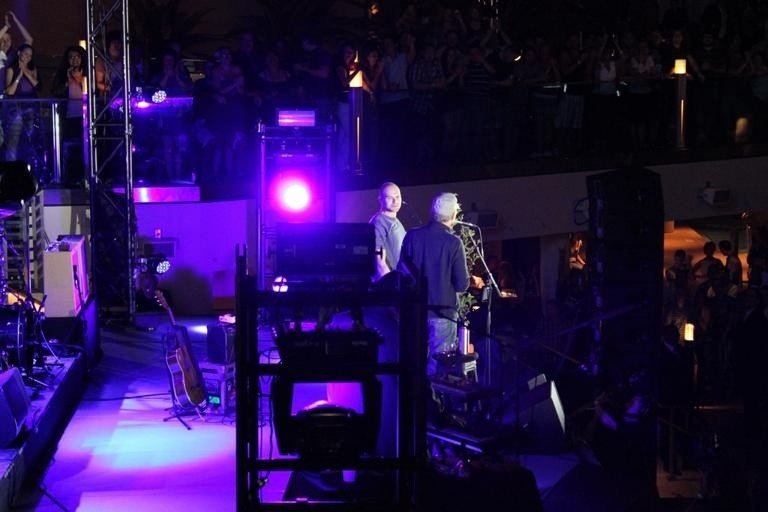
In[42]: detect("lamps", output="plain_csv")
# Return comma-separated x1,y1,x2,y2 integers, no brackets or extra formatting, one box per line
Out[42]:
138,79,167,104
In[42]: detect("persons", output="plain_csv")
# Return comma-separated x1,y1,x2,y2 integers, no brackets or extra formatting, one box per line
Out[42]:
667,226,768,434
560,268,592,323
369,181,406,277
396,192,472,376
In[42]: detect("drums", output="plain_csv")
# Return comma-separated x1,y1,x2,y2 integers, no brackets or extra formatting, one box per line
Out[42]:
1,303,34,380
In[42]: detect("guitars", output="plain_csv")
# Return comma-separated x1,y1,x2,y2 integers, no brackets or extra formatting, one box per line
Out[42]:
151,288,210,415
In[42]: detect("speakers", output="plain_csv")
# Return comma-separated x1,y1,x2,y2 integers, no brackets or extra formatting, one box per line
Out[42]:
585,167,664,315
0,367,30,447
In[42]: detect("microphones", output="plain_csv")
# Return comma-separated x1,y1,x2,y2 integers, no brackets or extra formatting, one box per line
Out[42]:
455,220,476,227
